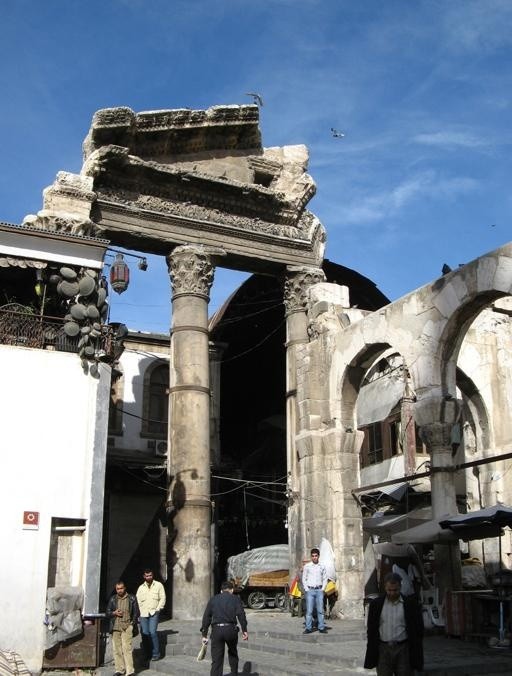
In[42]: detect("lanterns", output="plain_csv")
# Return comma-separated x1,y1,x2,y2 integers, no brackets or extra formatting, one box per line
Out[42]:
110,253,130,295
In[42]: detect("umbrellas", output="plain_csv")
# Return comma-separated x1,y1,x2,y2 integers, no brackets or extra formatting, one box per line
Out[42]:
370,542,430,567
395,513,505,589
363,504,433,558
439,500,511,639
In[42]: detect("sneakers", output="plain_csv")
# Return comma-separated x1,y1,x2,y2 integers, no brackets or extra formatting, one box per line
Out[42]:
319,629,328,633
152,654,160,661
303,629,311,634
113,672,121,676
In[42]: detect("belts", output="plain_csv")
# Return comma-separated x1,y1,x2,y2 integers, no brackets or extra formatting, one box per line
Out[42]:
216,623,231,627
308,587,321,589
382,641,407,646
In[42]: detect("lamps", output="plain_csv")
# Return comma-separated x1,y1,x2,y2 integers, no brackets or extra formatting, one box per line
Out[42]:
109,249,147,295
487,569,512,650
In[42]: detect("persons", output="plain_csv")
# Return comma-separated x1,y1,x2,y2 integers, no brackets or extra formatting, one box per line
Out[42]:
106,580,141,676
363,573,425,676
136,569,167,661
393,555,423,602
197,581,248,676
302,548,328,635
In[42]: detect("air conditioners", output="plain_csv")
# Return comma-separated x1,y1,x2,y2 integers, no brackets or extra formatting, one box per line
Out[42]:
153,440,167,456
